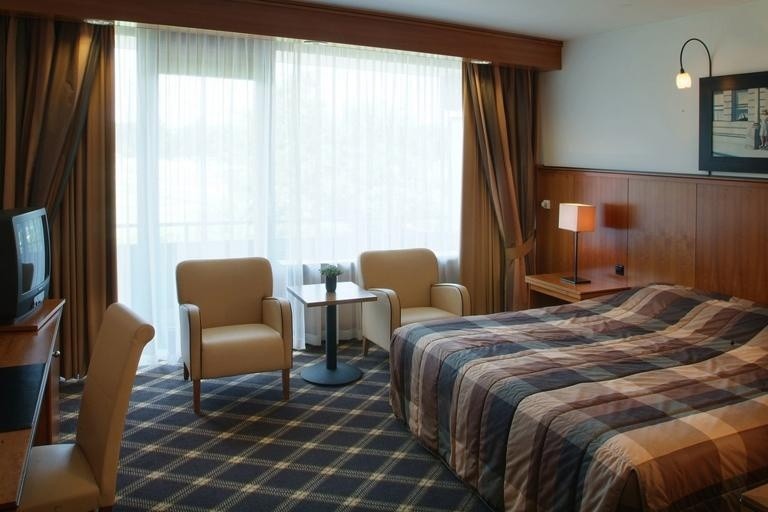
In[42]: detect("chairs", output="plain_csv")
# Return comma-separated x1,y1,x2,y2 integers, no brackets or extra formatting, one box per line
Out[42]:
13,301,156,512
174,257,293,414
356,247,472,356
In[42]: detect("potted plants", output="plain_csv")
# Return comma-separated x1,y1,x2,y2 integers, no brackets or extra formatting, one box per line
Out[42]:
318,265,343,291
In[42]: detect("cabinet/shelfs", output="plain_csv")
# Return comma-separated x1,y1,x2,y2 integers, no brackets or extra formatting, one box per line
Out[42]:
49,330,61,444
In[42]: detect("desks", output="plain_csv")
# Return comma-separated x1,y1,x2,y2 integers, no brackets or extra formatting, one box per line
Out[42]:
287,281,378,387
0,298,65,512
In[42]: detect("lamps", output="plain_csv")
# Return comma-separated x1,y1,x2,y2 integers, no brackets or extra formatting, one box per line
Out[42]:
675,38,712,88
558,203,595,284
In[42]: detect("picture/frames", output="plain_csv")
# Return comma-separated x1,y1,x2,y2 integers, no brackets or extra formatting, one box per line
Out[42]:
698,71,768,177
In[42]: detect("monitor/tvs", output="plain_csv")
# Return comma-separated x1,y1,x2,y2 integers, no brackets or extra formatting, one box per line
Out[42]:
0,207,52,326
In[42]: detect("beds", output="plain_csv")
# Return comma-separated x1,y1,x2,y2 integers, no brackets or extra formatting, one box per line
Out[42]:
387,282,768,512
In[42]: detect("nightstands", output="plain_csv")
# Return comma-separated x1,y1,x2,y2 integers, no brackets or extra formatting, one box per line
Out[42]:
525,272,632,310
